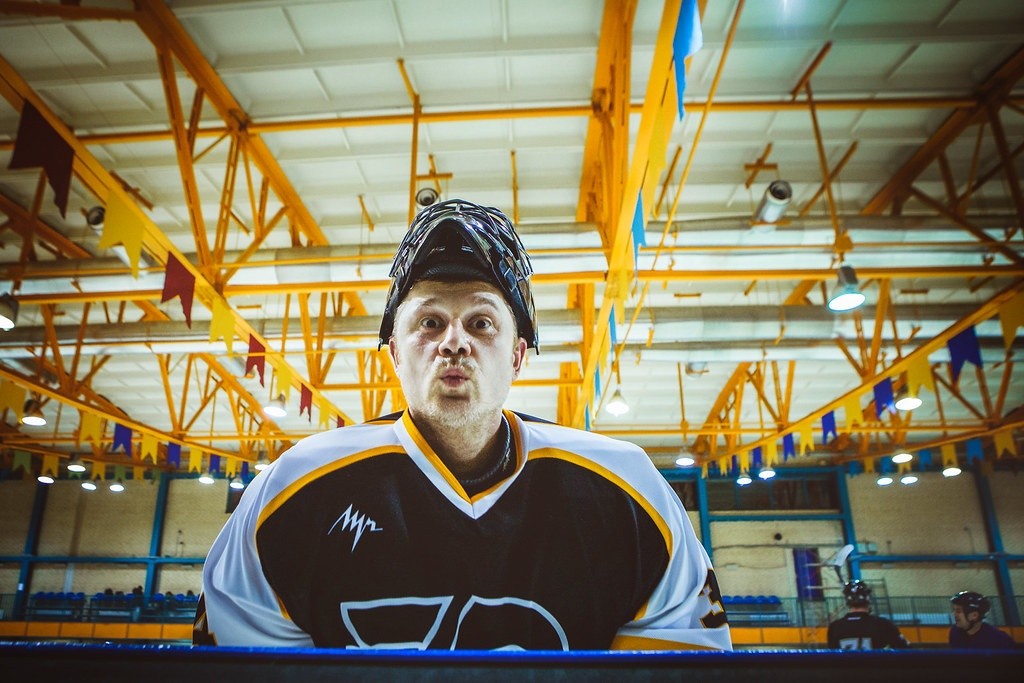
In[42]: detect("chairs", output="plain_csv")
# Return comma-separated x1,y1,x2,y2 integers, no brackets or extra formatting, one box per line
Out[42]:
721,595,784,613
30,591,200,610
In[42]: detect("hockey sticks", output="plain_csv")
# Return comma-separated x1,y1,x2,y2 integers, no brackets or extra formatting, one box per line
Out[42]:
830,543,855,585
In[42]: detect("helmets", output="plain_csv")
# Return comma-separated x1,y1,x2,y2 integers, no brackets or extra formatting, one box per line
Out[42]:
376,198,541,357
950,591,991,619
843,581,872,606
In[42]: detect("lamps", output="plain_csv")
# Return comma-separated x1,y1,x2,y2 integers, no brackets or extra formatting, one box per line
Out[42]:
828,261,865,311
896,385,925,411
605,390,630,416
255,459,270,470
675,446,694,466
67,460,86,472
110,481,125,491
37,473,56,484
737,471,753,485
80,480,97,491
263,395,287,417
198,467,214,484
230,478,245,489
758,464,776,479
21,402,47,426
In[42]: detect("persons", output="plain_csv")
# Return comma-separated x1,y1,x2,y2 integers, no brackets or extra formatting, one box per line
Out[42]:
947,590,1019,648
191,199,733,652
827,580,911,651
